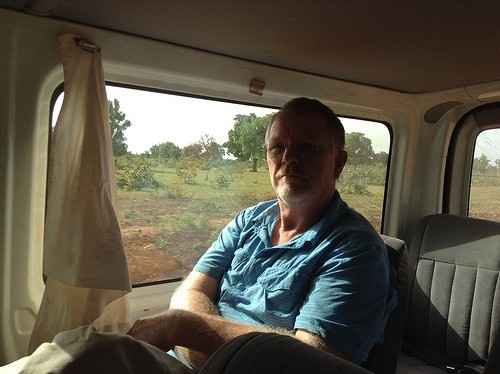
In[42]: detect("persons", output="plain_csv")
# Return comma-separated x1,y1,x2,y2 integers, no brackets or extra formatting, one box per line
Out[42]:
0,96,399,373
118,147,243,285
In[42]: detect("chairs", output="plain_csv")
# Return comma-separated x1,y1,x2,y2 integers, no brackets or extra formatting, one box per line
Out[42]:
397,213,500,374
379,234,410,374
